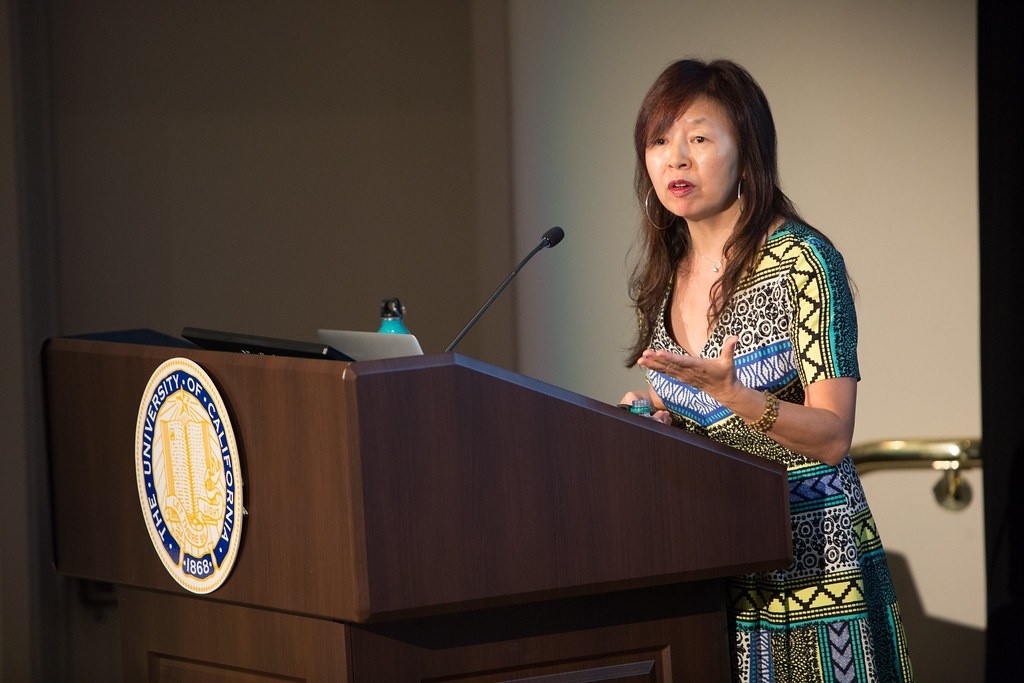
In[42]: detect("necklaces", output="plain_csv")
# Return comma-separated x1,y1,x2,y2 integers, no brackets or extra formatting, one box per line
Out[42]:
694,240,726,272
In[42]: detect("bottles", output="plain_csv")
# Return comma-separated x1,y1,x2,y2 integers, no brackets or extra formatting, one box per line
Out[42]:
630,401,662,423
375,298,409,334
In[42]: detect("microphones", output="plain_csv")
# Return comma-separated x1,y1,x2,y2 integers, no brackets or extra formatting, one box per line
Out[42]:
445,227,564,355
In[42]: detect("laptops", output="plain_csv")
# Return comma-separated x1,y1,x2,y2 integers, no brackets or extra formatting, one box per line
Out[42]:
181,326,355,363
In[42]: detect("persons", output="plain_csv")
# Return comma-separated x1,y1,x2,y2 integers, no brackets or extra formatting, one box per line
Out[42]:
619,58,914,683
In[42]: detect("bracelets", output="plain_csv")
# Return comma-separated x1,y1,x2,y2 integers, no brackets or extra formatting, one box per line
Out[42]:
743,389,780,437
